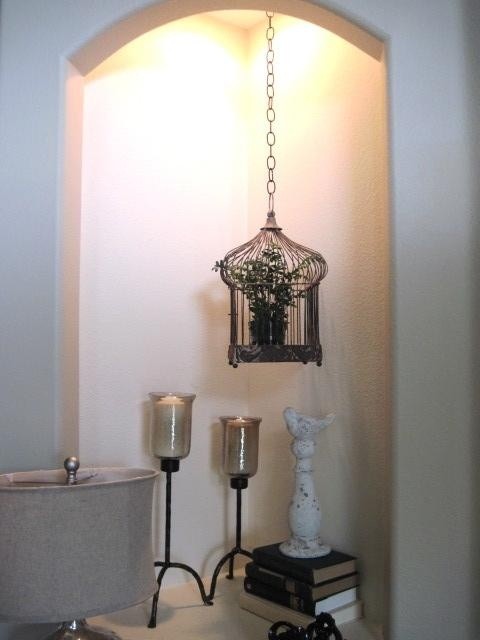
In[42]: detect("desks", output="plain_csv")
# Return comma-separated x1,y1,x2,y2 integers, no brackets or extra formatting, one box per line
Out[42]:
85,564,384,639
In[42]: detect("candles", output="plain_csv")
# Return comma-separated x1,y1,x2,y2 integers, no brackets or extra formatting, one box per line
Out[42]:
153,392,189,459
224,417,258,476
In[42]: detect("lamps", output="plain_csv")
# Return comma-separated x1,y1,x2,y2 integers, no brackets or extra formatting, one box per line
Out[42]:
1,456,160,640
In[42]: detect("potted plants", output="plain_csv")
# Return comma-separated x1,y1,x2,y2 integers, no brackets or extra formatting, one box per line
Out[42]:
213,245,325,344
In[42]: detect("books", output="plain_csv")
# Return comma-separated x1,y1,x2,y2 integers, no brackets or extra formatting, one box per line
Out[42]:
239,540,365,631
